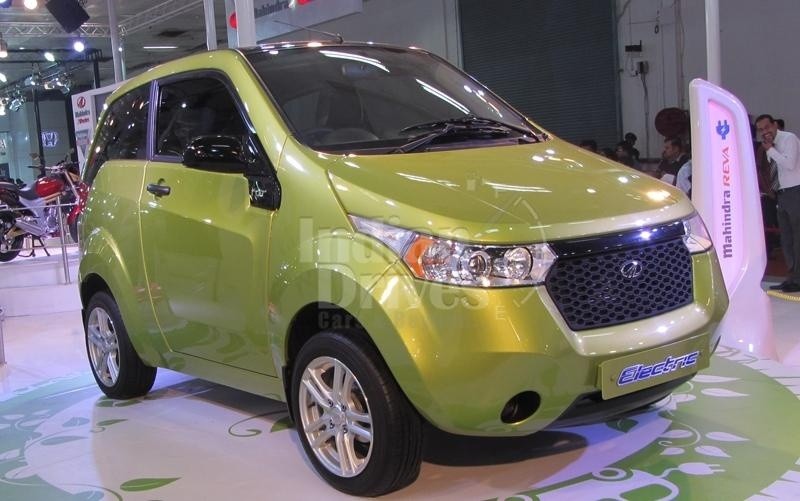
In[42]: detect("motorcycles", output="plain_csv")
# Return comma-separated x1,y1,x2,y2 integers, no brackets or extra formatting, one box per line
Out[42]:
0,148,83,262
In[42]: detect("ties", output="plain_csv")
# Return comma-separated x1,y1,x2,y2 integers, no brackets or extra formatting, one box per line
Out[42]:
768,144,780,192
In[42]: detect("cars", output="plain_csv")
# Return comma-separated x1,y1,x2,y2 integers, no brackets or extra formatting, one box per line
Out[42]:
75,38,731,500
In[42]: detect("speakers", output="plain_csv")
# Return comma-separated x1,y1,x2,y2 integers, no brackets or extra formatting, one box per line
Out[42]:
45,0,89,33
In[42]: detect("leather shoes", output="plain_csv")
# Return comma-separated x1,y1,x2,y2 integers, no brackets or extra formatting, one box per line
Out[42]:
770,281,800,292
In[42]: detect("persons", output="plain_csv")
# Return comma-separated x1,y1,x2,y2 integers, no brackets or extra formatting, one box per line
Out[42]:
580,132,640,171
750,114,800,295
657,136,693,199
0,174,44,189
